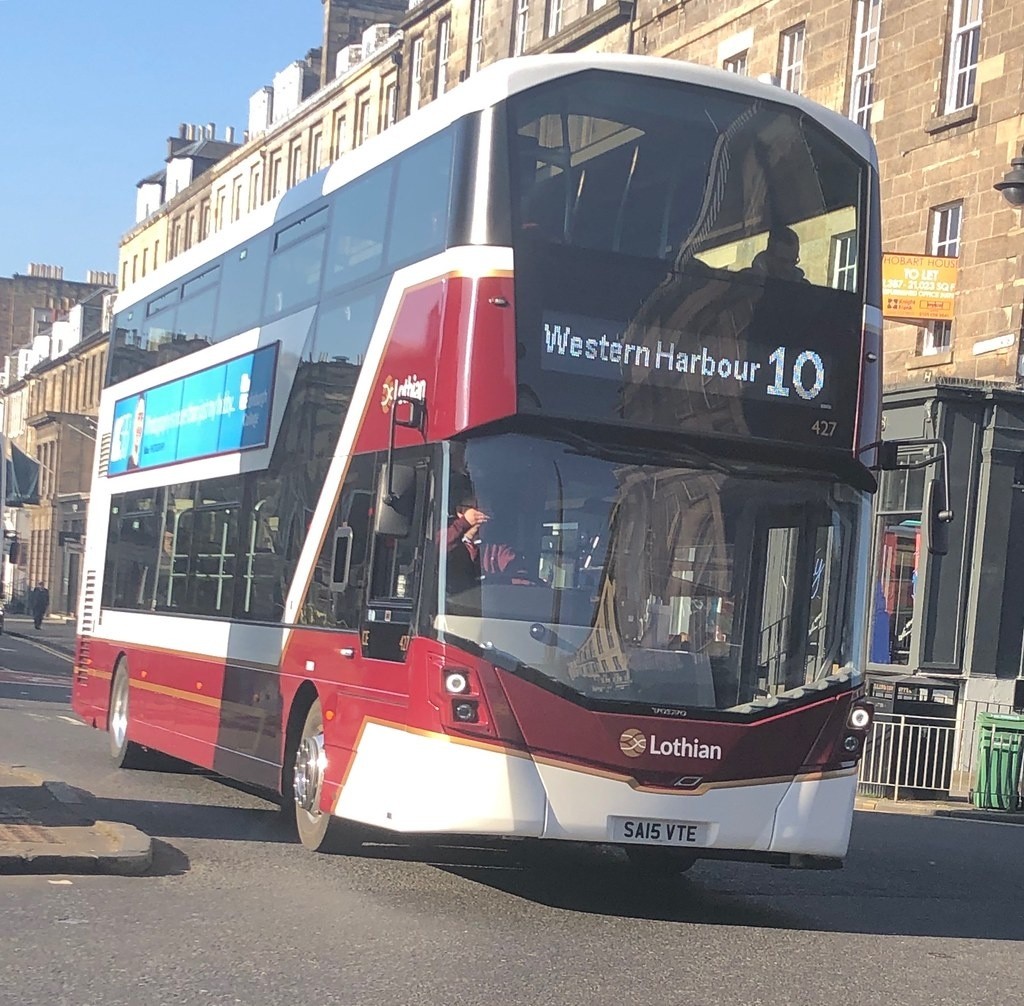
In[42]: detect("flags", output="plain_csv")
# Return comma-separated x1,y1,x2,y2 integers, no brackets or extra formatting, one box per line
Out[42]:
0,441,42,507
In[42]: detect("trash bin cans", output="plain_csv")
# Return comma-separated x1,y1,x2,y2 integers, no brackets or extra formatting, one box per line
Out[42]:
857,674,961,801
968,712,1024,811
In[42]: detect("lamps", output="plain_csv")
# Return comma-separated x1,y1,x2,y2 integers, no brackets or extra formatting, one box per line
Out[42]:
993,145,1024,209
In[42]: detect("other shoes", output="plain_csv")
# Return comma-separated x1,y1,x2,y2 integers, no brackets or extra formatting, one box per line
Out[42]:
35,625,40,630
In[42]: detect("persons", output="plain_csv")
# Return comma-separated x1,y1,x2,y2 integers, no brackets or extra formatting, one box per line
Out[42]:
435,488,539,587
152,520,202,608
32,582,50,631
736,224,811,284
126,394,146,470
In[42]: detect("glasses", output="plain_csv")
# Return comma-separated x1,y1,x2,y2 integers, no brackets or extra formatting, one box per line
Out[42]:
773,254,800,266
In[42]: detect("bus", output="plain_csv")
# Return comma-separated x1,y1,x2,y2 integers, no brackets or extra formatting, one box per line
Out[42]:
70,49,955,879
